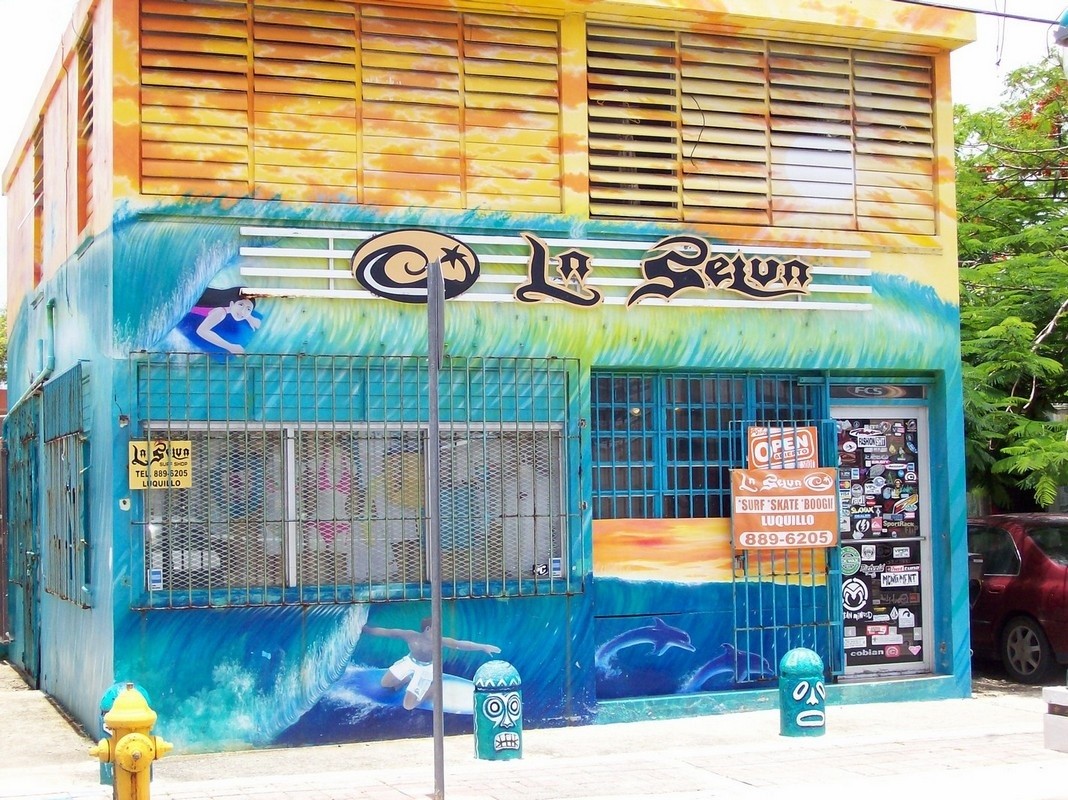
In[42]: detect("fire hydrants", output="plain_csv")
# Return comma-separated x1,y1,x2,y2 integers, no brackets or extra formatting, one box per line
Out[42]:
87,682,174,800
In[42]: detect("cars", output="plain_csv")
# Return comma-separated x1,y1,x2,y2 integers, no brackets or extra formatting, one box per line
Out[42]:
966,512,1068,685
